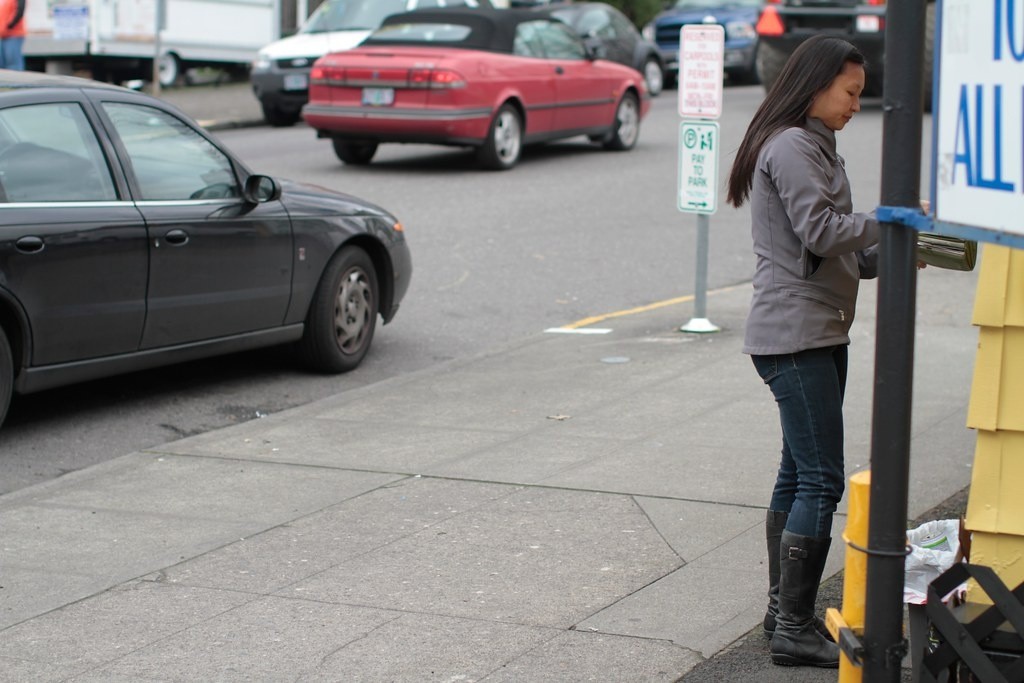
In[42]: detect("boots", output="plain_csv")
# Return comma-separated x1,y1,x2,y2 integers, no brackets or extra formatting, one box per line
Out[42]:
763,508,835,641
770,529,840,669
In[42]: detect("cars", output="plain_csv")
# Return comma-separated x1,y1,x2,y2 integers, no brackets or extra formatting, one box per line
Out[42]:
0,72,412,426
640,0,764,83
250,1,511,125
300,6,649,170
518,0,666,100
754,0,888,95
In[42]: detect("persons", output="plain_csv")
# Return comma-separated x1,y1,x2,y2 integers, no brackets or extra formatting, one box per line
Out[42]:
726,34,929,668
0,0,26,70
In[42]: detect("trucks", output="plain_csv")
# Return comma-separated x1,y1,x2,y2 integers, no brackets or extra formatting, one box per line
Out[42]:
21,0,282,90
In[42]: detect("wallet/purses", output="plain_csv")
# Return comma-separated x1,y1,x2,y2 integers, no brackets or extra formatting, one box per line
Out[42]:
918,231,978,272
